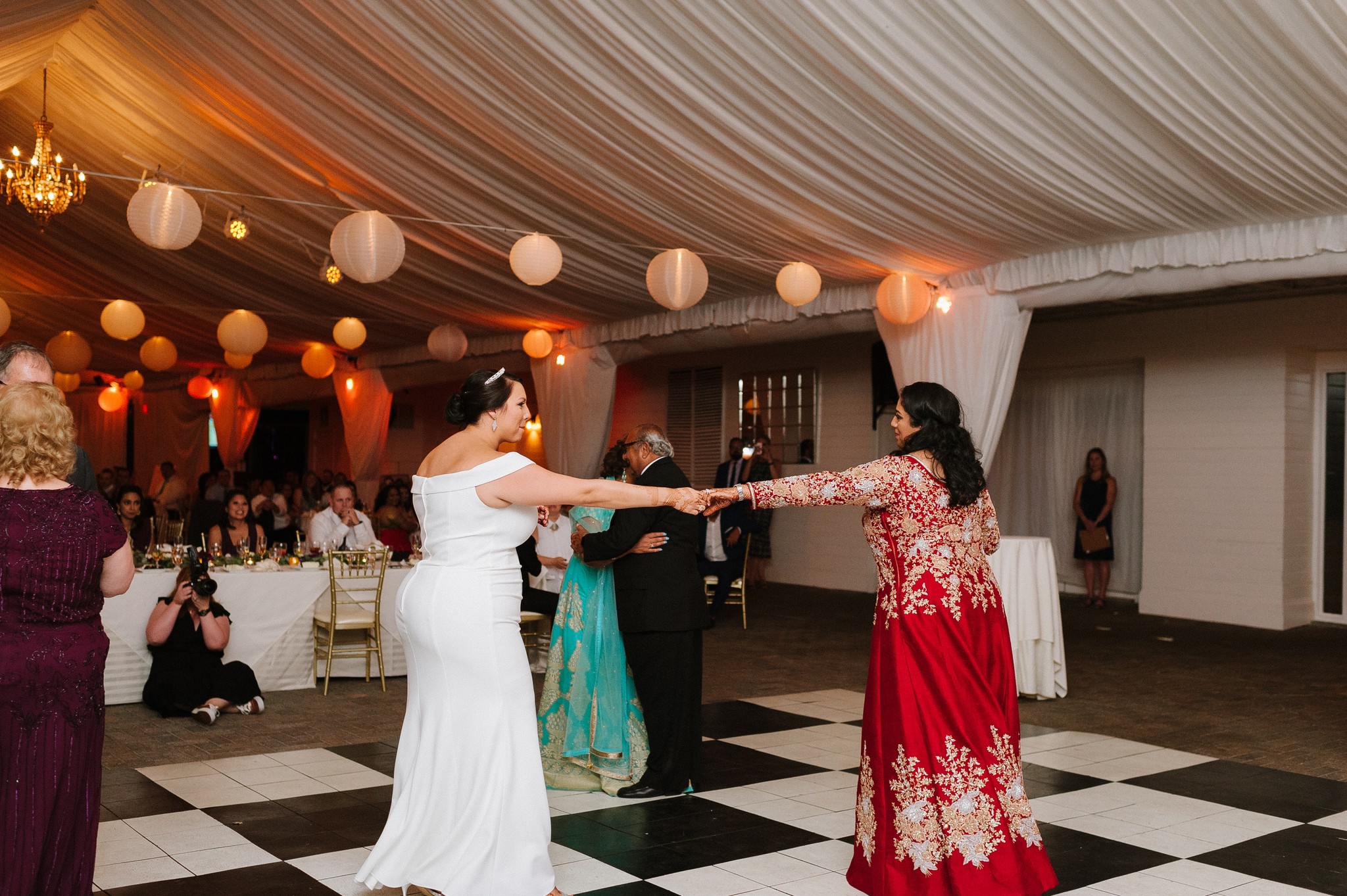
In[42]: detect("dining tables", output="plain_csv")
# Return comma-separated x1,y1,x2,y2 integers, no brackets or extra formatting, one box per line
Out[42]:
98,550,430,707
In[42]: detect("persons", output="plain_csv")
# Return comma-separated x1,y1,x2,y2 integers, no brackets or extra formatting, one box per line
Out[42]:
571,420,705,799
698,382,1061,896
0,461,452,561
535,434,696,796
0,385,135,896
742,434,782,593
0,341,99,491
713,437,753,580
142,564,268,728
797,438,815,465
1072,446,1119,611
693,498,748,633
353,363,707,896
510,528,541,634
526,501,578,635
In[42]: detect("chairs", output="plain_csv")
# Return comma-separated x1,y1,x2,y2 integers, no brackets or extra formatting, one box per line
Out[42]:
311,545,388,696
147,514,187,553
700,530,750,631
516,609,547,663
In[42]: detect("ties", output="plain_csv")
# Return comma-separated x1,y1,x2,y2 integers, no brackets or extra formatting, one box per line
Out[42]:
337,534,347,553
158,480,169,495
728,461,738,488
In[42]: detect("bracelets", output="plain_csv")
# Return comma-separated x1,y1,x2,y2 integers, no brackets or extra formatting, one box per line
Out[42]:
1093,519,1100,527
611,557,617,560
767,460,775,465
733,482,745,502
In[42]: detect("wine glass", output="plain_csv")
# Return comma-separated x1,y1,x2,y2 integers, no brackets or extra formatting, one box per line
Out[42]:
172,548,183,571
152,551,162,570
236,536,250,569
258,536,266,563
210,542,219,557
410,533,420,554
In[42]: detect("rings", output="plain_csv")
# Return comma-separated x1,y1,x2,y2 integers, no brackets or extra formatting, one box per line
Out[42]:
651,543,654,549
705,487,710,495
697,504,703,512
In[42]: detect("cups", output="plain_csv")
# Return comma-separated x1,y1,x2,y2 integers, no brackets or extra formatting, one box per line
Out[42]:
293,541,306,555
269,542,287,561
308,541,320,553
322,538,338,553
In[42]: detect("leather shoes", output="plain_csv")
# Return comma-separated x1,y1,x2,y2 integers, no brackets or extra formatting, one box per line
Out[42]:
617,780,689,800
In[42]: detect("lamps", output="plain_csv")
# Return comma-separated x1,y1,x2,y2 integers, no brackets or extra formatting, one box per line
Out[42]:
317,254,344,285
0,60,90,240
222,205,249,240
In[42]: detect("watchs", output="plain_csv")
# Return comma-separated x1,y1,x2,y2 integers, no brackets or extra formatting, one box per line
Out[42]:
197,608,211,616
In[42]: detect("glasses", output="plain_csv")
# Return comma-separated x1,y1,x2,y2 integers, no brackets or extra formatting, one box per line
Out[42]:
730,444,743,450
620,440,645,454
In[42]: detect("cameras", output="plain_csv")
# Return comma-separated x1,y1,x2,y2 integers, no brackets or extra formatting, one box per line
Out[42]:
182,546,218,600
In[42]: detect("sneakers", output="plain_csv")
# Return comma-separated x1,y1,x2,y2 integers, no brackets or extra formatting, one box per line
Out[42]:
234,696,265,716
191,703,220,726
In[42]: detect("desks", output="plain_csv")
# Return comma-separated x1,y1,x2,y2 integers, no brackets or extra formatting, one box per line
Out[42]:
982,535,1070,703
